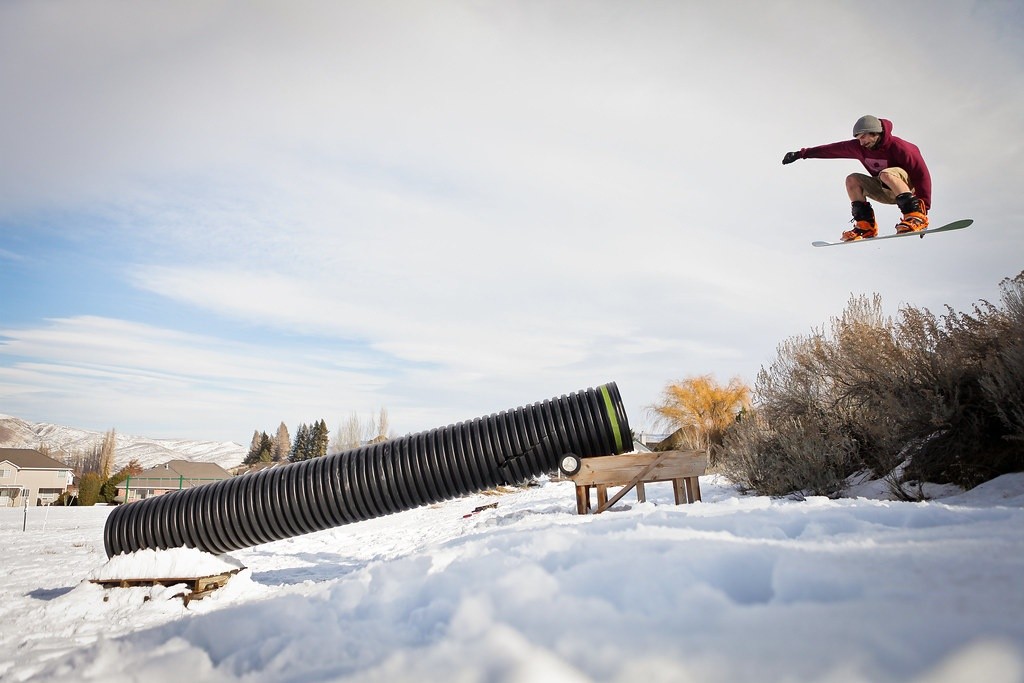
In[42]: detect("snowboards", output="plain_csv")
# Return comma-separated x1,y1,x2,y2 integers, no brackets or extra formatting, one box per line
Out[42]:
811,218,974,248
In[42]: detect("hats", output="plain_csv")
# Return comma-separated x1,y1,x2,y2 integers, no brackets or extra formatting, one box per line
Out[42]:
853,115,883,136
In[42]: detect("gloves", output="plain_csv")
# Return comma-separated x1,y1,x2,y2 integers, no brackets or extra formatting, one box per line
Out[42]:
920,211,928,238
782,151,800,165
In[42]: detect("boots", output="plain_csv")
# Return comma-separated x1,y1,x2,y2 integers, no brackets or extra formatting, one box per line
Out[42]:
894,192,929,234
841,201,878,242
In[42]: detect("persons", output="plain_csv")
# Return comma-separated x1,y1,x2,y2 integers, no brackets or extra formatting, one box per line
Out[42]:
782,115,932,242
